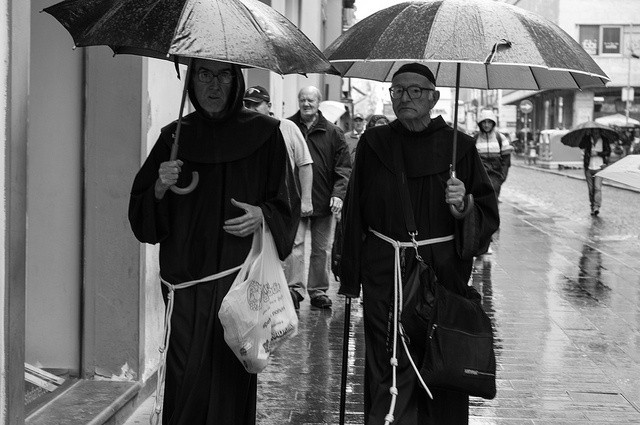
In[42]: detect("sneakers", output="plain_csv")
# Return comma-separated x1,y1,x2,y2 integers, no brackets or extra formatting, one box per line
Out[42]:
290,289,303,309
590,208,595,215
594,207,599,214
310,296,332,308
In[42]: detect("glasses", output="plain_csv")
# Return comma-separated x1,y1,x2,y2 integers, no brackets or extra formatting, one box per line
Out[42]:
197,67,233,84
389,83,434,99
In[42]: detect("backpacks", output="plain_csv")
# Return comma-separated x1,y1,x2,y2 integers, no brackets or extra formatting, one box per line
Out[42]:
401,255,496,399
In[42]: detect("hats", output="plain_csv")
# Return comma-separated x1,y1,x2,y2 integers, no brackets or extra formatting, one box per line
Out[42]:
392,62,436,86
243,85,269,102
352,113,363,120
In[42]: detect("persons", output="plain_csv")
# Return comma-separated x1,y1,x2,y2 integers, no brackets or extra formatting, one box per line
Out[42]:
125,58,301,420
335,61,501,425
469,108,512,256
366,114,391,128
242,85,316,219
284,85,353,309
526,141,537,166
619,128,634,156
343,112,366,167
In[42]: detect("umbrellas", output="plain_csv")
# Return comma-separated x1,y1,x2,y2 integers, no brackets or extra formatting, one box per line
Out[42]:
37,0,327,82
318,98,346,124
323,1,612,96
592,153,640,191
